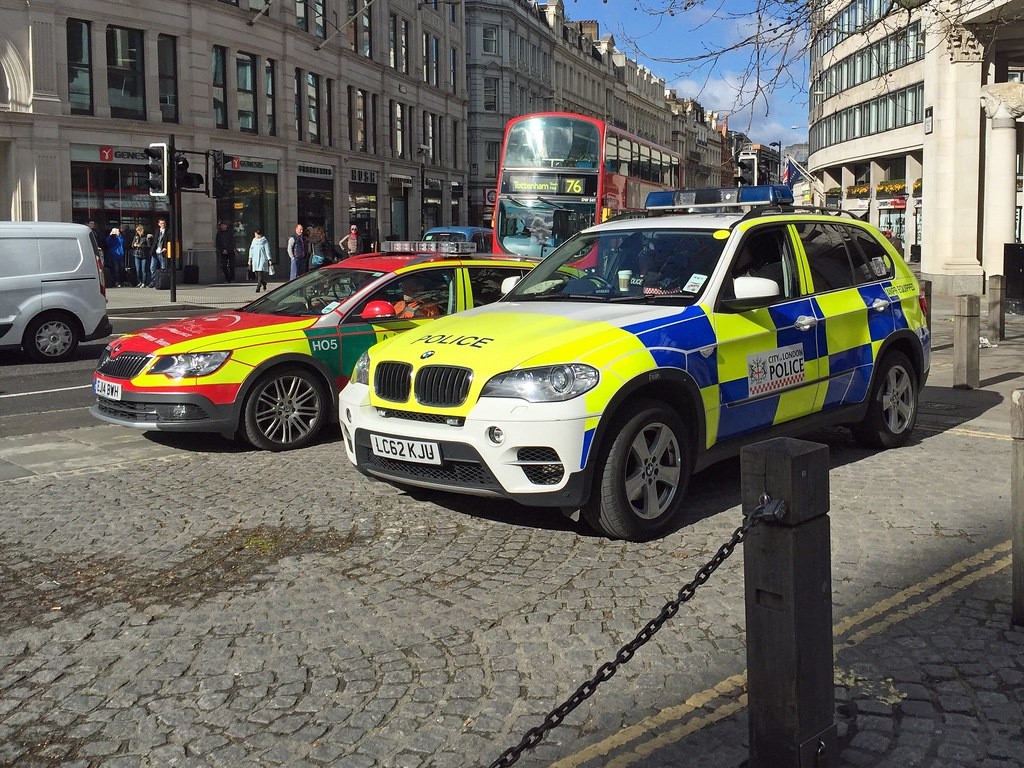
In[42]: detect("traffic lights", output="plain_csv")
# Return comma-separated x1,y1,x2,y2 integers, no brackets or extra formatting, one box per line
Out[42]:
176,157,190,188
144,142,168,196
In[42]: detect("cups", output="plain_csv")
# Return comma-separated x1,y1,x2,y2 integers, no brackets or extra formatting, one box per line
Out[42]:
618,270,633,291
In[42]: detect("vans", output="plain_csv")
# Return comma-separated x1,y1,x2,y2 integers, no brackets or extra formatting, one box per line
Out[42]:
0,220,113,364
422,226,493,253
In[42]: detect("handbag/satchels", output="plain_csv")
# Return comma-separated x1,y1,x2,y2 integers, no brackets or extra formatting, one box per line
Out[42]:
269,265,277,275
312,255,324,265
246,266,254,281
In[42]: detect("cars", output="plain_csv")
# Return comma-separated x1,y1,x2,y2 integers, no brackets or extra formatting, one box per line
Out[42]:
89,240,497,448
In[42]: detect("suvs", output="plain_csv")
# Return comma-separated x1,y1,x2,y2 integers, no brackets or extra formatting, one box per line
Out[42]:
337,185,931,540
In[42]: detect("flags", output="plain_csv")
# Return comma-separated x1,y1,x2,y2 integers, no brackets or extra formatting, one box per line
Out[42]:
782,158,801,189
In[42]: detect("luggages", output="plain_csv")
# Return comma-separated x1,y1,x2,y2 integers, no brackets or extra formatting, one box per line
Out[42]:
156,250,171,289
184,249,199,284
120,249,137,287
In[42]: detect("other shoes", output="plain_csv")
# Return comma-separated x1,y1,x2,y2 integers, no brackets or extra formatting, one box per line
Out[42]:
146,285,152,288
118,285,121,287
135,282,142,287
256,288,260,293
223,280,230,283
140,284,145,288
230,280,234,283
264,282,268,290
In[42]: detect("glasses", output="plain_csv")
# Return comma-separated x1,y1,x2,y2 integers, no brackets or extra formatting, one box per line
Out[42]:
351,228,357,230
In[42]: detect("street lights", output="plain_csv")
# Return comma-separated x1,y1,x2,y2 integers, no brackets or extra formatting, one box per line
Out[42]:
738,157,757,187
769,141,781,184
416,144,430,240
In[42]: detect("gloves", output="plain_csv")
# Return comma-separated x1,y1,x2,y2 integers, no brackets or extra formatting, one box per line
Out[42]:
344,249,351,251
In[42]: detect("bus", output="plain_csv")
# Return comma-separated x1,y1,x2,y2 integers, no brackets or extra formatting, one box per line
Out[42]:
488,110,682,276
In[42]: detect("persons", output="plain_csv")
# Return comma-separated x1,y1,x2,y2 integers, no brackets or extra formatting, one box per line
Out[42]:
146,218,169,290
216,220,236,284
106,228,126,286
306,225,331,273
132,225,148,288
87,219,99,249
248,230,273,293
339,224,365,259
392,276,440,318
286,225,306,281
981,83,1024,119
